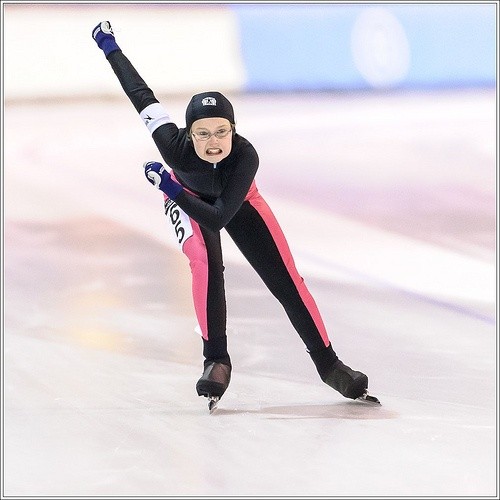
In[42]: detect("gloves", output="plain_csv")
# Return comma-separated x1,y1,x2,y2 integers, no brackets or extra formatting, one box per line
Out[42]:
143,161,184,200
91,21,122,60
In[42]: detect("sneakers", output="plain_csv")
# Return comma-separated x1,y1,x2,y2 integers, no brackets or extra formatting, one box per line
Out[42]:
195,336,232,415
304,342,382,408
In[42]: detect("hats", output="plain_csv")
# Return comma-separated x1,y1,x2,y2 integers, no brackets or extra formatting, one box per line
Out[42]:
185,92,235,129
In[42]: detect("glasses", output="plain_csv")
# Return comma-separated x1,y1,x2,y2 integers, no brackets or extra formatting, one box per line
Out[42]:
190,125,234,140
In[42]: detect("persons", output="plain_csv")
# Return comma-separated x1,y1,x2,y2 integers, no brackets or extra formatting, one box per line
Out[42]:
92,20,370,401
